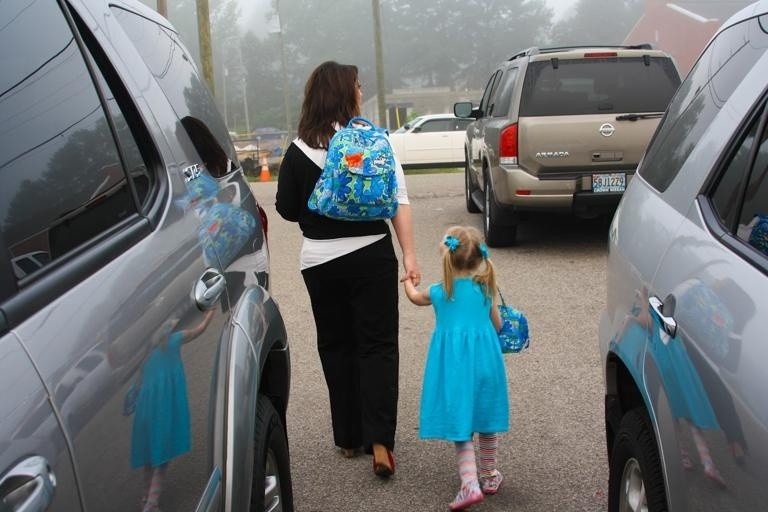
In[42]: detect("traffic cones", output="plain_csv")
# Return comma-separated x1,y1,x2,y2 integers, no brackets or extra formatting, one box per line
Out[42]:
257,155,275,182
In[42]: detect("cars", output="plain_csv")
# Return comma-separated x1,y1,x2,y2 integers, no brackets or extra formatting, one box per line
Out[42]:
387,110,471,170
252,127,287,141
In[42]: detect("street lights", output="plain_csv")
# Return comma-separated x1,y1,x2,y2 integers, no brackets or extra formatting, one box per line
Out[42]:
223,65,251,138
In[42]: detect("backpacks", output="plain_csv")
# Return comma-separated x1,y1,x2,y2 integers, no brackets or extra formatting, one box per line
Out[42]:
185,170,258,271
306,116,398,225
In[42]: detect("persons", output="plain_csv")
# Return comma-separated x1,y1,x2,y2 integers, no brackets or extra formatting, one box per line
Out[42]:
130,308,215,512
401,224,508,511
647,320,727,489
174,116,268,313
684,277,757,466
274,62,422,474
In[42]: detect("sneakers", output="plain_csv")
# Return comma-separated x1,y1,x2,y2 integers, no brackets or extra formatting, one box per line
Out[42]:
448,482,484,511
480,467,503,494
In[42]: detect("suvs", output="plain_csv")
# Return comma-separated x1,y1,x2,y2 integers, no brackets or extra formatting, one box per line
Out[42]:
47,340,122,452
0,0,303,512
596,4,768,510
453,42,682,250
5,244,51,283
107,237,213,377
42,164,152,261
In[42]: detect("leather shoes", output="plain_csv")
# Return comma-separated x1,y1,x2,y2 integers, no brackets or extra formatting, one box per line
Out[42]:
341,441,395,479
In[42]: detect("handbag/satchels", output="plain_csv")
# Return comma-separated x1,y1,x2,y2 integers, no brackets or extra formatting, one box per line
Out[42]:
491,284,531,355
124,380,139,418
